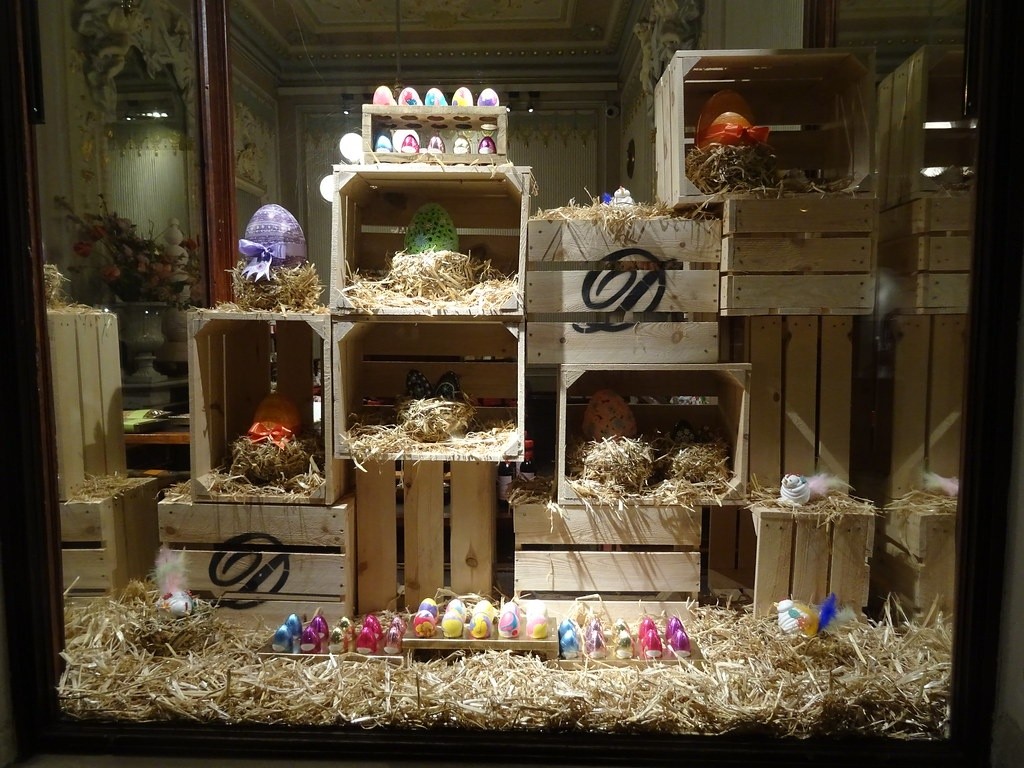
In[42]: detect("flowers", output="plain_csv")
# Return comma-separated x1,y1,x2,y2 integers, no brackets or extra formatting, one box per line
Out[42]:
51,191,200,310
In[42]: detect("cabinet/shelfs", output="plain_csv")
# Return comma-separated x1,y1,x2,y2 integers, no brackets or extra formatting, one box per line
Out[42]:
122,426,190,487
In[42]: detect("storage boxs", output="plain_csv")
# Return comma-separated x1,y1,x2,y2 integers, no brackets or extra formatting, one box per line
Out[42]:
61,477,160,608
185,310,354,506
158,484,357,629
355,458,498,616
728,314,854,499
853,315,972,509
362,103,508,166
706,506,875,621
875,42,966,213
876,507,958,631
721,197,876,316
656,43,881,211
331,313,524,462
526,218,721,365
43,309,127,500
329,163,532,316
512,502,704,622
555,362,750,506
878,195,975,315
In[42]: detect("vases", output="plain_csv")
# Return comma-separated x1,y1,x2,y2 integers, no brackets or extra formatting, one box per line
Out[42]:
116,302,168,383
